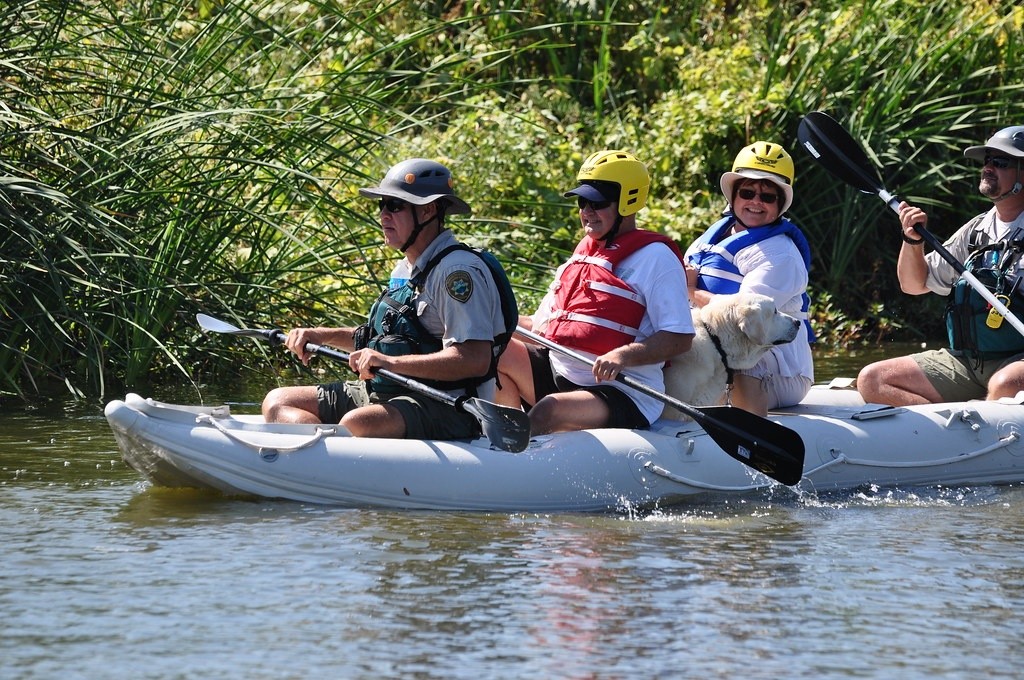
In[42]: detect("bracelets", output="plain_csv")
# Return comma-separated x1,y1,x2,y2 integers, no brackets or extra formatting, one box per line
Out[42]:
901,229,925,245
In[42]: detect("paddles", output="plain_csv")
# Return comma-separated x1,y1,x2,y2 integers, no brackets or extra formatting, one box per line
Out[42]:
797,111,1024,338
513,323,806,487
194,309,531,454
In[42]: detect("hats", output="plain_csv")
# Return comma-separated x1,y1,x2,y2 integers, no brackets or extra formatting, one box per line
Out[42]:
564,181,620,202
720,169,794,217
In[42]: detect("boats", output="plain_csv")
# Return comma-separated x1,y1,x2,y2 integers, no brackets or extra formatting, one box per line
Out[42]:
103,379,1023,518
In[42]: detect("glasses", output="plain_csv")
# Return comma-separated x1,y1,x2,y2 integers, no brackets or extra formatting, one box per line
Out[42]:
735,189,778,204
577,195,611,210
378,198,404,212
984,154,1016,170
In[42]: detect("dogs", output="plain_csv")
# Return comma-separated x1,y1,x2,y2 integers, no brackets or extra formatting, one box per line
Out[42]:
659,292,801,422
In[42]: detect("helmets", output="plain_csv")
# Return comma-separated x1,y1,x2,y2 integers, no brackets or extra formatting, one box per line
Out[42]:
720,141,795,217
964,126,1024,160
563,150,650,216
358,158,471,215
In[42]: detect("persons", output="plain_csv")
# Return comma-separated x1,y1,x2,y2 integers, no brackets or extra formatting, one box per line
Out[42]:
261,159,519,441
683,139,815,417
857,125,1024,406
496,150,697,437
